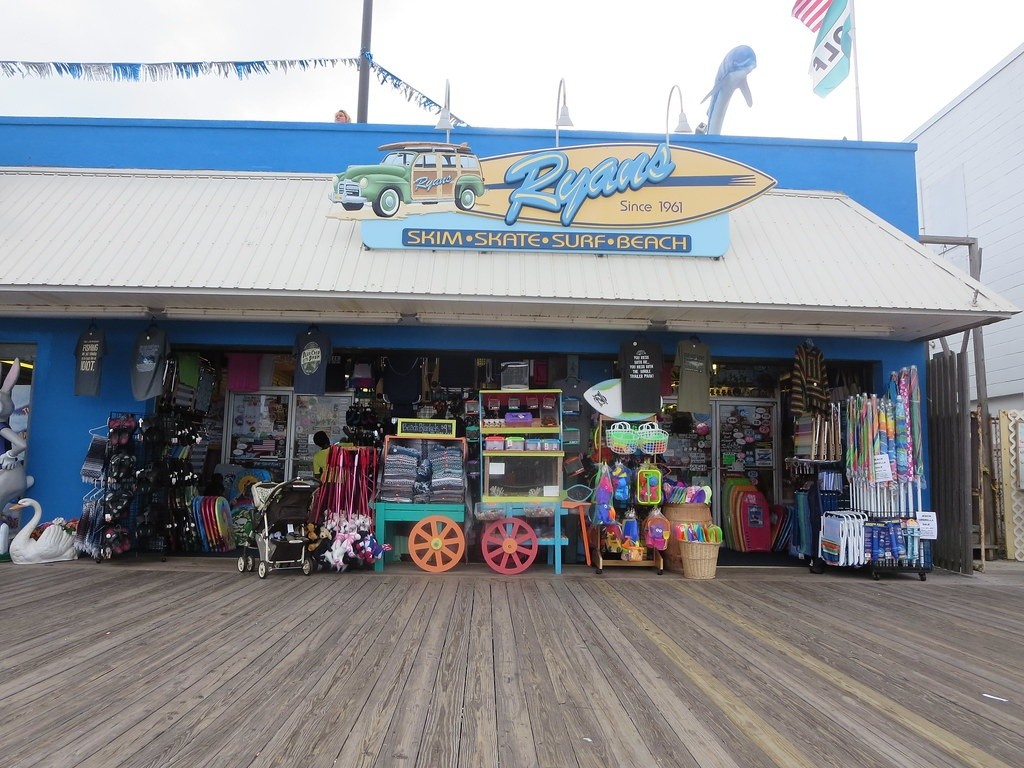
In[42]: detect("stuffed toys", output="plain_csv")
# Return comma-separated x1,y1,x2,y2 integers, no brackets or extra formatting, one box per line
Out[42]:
298,509,394,574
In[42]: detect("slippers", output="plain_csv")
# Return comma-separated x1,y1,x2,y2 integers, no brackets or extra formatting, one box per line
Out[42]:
102,406,205,562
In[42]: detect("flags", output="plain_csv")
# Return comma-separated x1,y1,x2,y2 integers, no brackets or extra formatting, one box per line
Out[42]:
251,439,276,452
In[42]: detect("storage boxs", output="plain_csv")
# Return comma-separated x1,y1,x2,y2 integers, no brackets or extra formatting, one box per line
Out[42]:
664,502,711,572
678,539,723,580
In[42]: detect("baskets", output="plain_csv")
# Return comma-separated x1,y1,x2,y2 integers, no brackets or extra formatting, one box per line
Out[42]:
637,422,669,455
605,422,639,455
678,539,724,580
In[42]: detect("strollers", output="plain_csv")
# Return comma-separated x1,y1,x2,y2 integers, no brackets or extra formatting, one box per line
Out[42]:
237,476,323,578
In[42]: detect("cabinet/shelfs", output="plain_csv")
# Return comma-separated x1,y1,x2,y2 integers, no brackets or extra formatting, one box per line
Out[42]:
375,418,468,572
478,388,569,574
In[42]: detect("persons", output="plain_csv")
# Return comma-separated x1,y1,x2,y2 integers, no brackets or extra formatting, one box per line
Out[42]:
310,429,357,484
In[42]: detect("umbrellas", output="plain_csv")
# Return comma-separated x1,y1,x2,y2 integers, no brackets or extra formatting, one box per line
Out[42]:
842,362,929,520
